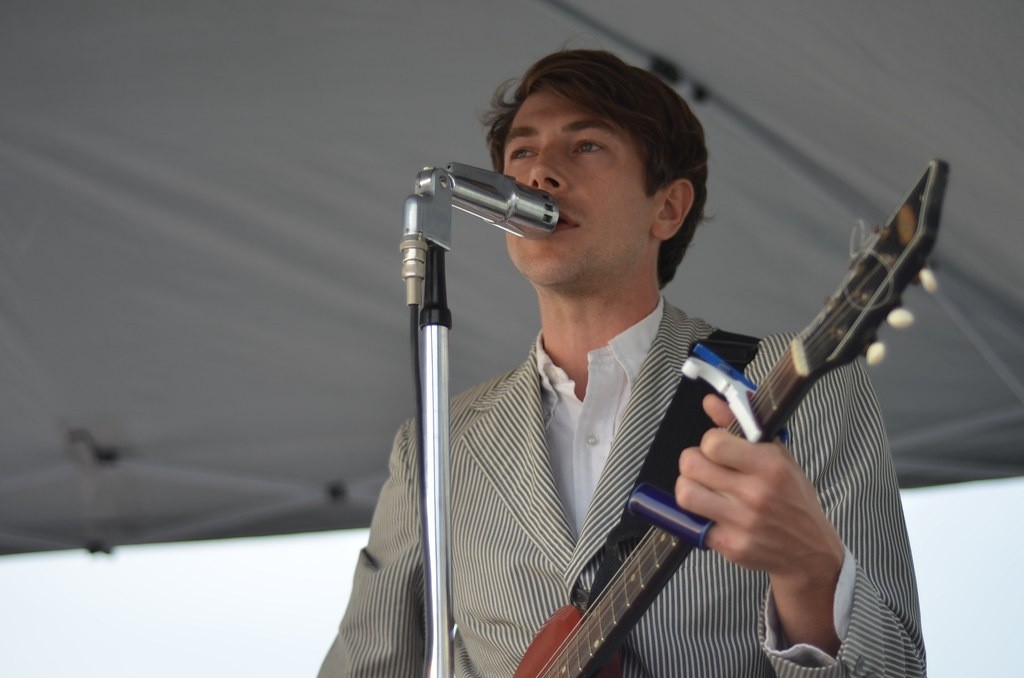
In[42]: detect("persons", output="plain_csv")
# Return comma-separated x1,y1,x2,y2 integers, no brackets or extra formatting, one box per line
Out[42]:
316,47,929,677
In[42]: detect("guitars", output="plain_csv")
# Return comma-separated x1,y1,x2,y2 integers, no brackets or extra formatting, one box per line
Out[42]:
530,159,954,678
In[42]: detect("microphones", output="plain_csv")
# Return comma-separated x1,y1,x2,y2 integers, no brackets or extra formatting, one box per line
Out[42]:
440,160,560,242
